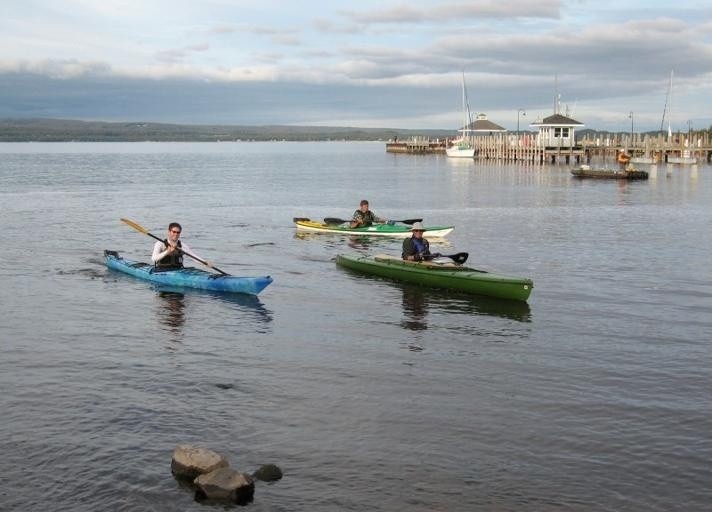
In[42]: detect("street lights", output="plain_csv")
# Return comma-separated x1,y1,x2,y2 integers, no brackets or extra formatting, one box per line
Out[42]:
472,112,478,130
517,109,526,134
629,111,634,147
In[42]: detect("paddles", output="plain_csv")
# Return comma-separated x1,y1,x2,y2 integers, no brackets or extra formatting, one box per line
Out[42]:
322,216,424,225
119,217,229,277
407,251,470,263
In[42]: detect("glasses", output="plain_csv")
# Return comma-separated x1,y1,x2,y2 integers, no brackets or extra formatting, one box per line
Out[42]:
172,230,180,234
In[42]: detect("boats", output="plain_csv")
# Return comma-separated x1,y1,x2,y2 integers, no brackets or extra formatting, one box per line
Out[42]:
293,217,455,238
336,253,533,302
570,169,649,180
104,249,274,296
445,145,482,157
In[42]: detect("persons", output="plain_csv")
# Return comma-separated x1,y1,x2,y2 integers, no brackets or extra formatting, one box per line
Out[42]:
351,200,395,229
151,222,213,272
402,221,441,261
618,149,631,169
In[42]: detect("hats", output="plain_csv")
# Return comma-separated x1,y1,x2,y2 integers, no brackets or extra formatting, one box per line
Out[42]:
410,222,426,232
